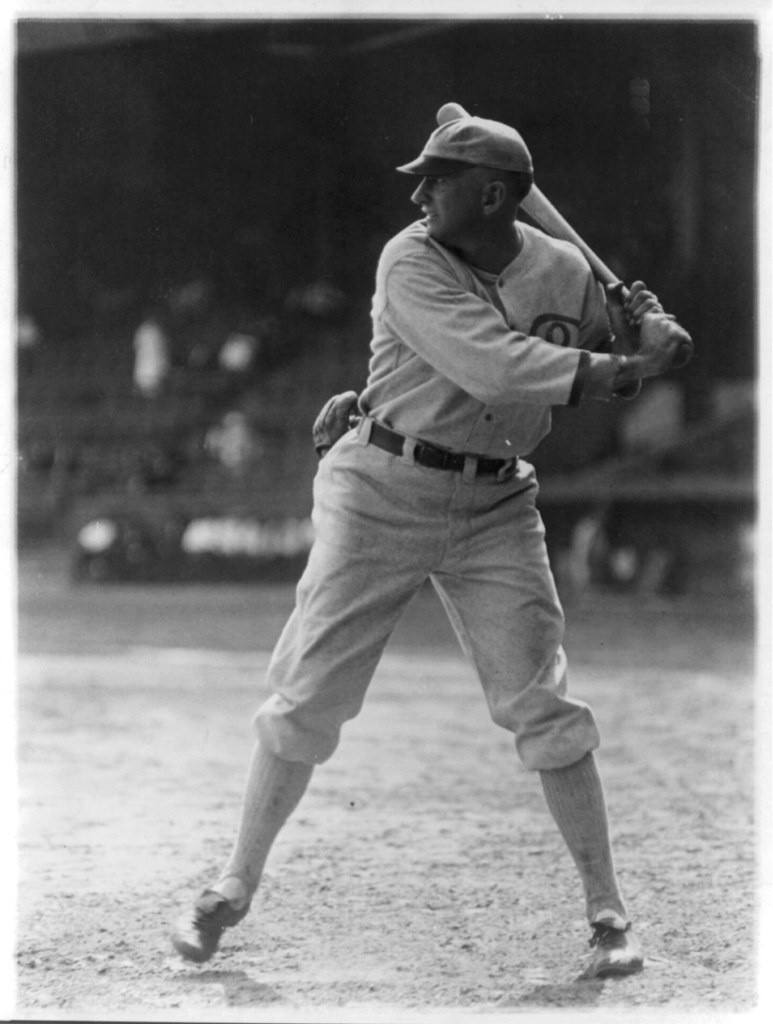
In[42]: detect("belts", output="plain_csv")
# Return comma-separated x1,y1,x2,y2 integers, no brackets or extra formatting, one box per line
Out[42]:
353,417,519,477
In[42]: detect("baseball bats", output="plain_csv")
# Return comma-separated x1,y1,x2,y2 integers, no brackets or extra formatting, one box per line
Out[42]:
435,102,693,369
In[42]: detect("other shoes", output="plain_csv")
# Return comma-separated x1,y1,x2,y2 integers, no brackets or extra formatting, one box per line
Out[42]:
589,909,644,977
174,900,225,962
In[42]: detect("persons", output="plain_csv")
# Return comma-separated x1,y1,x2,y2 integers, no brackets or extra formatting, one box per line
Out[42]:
168,99,698,982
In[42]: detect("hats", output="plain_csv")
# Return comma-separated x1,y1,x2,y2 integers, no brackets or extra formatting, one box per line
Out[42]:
394,116,535,176
